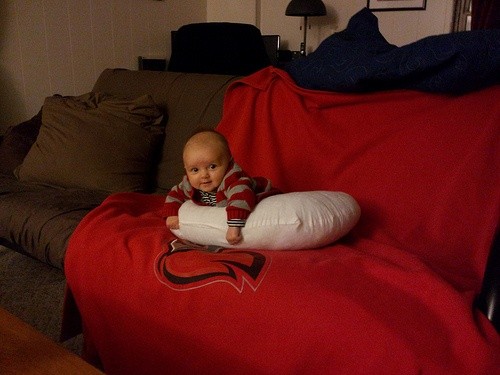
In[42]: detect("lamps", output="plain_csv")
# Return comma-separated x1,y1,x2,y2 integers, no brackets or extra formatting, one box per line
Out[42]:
285,0,328,58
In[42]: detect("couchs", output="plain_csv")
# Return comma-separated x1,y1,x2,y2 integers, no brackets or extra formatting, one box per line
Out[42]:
0,67,500,375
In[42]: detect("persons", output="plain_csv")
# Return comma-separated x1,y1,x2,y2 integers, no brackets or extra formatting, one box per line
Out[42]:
165,130,283,244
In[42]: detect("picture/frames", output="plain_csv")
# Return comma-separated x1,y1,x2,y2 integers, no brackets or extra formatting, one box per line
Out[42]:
367,0,426,12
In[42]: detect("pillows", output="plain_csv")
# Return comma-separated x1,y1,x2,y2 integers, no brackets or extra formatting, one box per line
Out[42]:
14,96,169,196
170,190,361,251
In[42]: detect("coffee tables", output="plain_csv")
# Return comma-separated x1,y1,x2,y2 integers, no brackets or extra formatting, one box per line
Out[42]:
0,306,106,375
